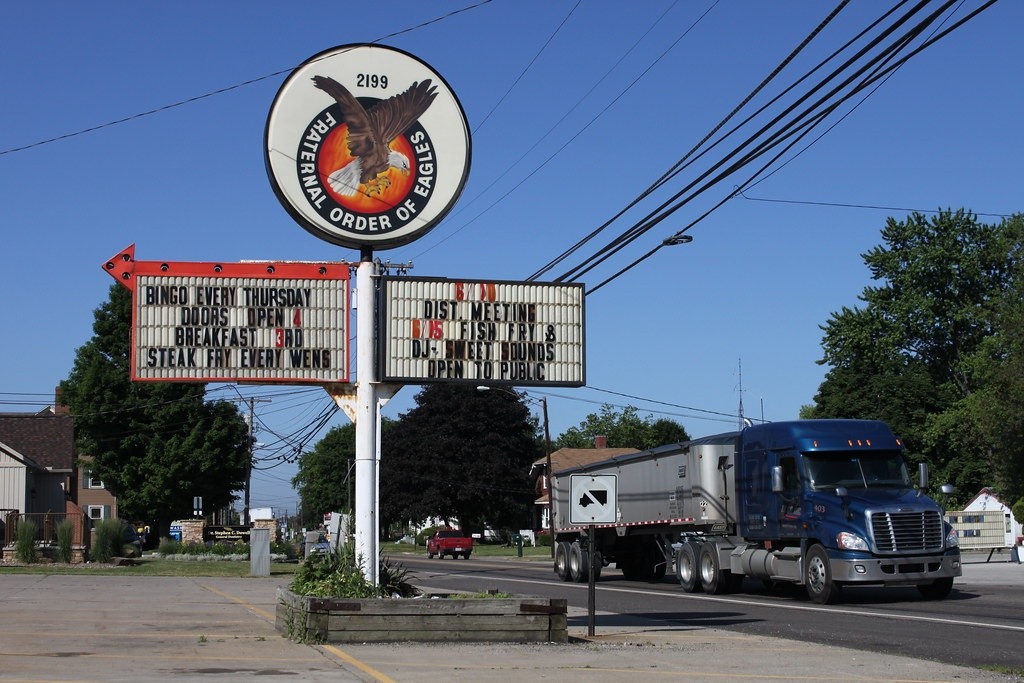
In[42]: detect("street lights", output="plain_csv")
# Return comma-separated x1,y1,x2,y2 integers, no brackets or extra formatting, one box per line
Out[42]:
476,385,555,558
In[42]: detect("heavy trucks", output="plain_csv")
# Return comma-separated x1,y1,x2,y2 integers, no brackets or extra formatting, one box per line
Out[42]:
547,417,965,603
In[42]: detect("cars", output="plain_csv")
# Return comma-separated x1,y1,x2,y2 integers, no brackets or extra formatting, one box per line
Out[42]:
298,534,330,557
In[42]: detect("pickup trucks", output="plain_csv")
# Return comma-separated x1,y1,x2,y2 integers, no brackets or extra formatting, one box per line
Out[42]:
426,529,473,560
